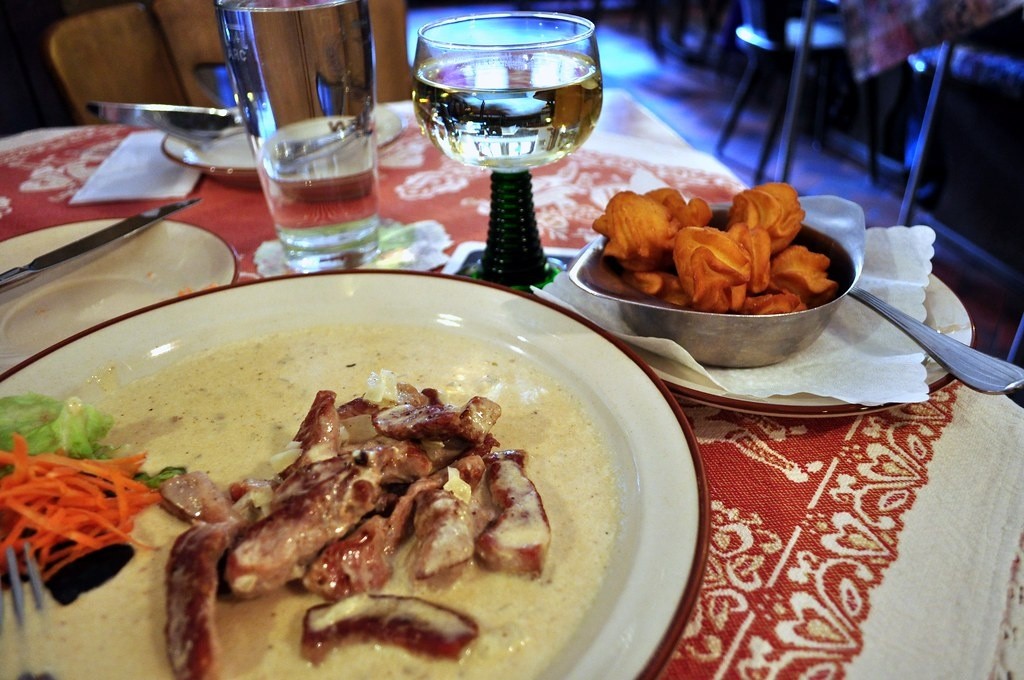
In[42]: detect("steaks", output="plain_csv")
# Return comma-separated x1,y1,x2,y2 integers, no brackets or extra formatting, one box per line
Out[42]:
159,385,552,680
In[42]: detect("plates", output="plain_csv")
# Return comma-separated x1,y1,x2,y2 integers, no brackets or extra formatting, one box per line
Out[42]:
618,271,977,417
1,262,711,680
0,218,241,377
160,107,406,183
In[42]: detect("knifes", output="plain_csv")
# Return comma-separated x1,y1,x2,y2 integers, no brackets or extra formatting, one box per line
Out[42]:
1,198,203,285
85,100,246,137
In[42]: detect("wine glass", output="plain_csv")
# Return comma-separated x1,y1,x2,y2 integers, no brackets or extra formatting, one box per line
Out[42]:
413,13,601,287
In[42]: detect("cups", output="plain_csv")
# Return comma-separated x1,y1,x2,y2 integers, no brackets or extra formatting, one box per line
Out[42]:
213,0,377,259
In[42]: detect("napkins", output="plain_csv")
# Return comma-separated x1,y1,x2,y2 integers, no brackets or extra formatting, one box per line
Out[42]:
530,220,936,409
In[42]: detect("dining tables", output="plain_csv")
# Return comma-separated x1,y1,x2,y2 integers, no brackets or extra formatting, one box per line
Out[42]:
3,91,1020,680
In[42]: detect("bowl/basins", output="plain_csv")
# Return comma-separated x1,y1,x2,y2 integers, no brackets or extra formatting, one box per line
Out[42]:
568,194,865,369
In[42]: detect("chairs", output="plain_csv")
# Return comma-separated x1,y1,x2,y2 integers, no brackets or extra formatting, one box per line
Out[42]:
47,0,414,128
712,0,879,193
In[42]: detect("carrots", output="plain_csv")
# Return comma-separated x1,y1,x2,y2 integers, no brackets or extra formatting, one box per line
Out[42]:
0,434,161,584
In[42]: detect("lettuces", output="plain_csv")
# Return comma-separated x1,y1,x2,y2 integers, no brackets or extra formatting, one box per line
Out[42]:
0,392,131,459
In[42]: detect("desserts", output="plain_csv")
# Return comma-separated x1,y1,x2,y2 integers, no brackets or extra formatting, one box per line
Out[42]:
593,179,838,314
